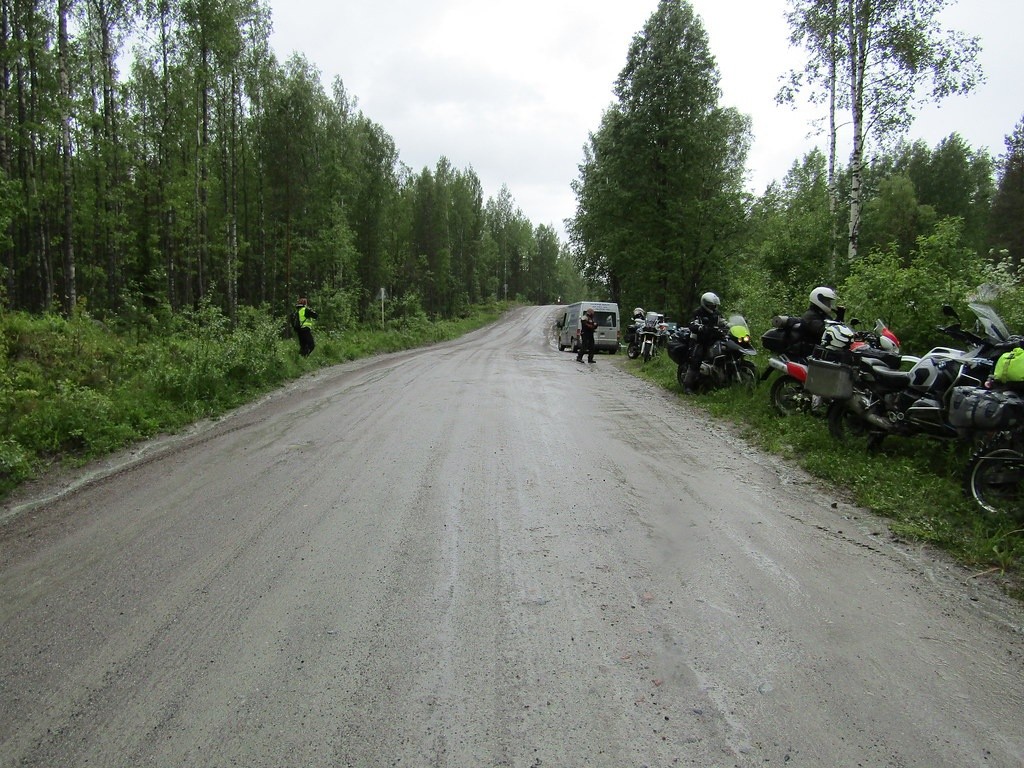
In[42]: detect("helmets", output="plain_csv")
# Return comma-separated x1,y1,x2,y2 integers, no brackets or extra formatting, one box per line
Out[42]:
701,292,720,313
809,287,836,314
821,325,854,348
634,308,644,316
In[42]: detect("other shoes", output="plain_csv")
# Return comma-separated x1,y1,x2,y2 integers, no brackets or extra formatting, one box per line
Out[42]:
588,358,596,363
577,356,584,363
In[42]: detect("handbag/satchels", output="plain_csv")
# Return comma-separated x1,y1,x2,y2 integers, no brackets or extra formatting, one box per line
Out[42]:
948,385,1024,430
994,347,1024,383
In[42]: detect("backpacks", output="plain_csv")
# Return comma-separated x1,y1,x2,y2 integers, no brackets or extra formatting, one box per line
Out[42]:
290,306,308,332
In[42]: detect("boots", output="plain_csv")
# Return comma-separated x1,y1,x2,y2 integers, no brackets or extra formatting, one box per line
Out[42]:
682,370,699,395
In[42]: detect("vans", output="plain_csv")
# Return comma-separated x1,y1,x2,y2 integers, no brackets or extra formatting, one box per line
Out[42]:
557,301,621,355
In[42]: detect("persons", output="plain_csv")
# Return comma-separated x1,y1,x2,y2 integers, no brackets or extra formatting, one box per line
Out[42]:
795,286,871,362
687,292,736,383
294,298,319,357
577,308,598,363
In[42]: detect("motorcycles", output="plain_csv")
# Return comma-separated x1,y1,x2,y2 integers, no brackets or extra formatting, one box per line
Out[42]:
950,335,1024,514
759,316,902,418
665,315,760,396
802,302,1024,454
627,312,670,364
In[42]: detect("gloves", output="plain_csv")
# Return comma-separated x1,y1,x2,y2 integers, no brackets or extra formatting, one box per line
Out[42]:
831,306,846,320
